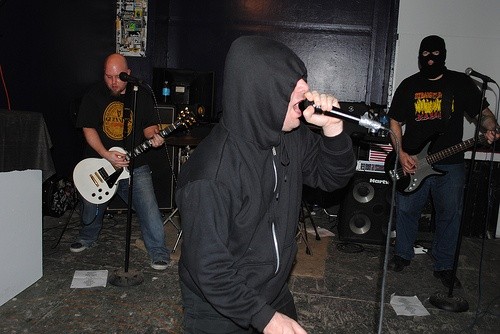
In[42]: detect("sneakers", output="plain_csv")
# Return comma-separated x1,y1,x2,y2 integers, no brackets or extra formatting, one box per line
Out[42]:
70,240,98,253
150,258,168,270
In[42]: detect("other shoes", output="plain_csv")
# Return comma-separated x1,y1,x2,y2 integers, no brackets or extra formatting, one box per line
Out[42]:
433,270,463,292
387,255,411,273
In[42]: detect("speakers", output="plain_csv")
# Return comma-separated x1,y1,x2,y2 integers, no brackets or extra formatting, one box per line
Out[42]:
340,172,398,246
106,105,175,212
152,68,215,123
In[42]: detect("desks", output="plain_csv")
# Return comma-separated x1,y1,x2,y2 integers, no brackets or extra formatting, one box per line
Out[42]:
464,144,500,238
0,109,55,308
168,135,201,175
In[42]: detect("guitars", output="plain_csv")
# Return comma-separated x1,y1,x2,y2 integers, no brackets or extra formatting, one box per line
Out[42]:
384,127,500,195
72,104,208,205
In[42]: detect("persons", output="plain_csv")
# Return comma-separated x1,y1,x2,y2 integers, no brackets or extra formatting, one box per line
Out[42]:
69,54,171,270
387,34,500,289
178,35,358,334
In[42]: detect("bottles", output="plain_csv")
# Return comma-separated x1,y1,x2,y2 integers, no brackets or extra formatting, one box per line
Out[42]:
162,80,171,106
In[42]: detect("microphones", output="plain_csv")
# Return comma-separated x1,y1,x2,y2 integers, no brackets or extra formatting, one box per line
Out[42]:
465,67,496,86
299,99,381,130
119,72,145,87
